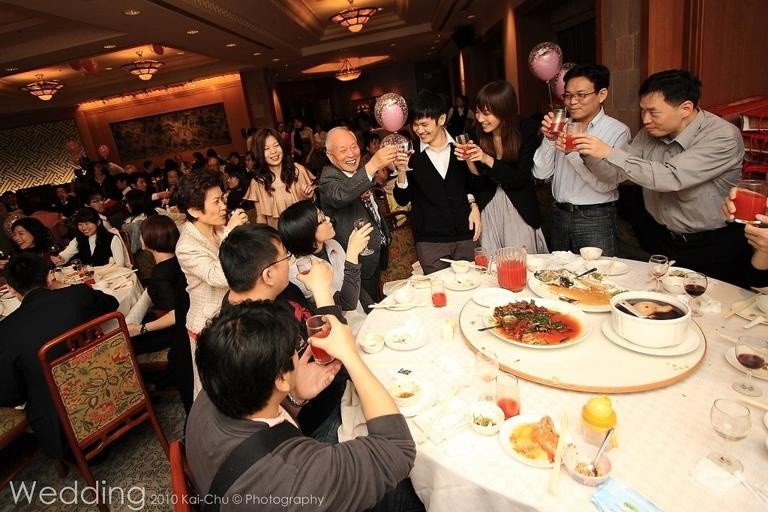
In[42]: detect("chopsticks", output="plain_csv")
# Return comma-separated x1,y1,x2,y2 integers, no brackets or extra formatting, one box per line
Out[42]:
367,304,431,309
416,379,458,445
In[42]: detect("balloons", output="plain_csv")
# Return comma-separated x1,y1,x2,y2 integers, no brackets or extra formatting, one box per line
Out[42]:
528,42,563,83
550,63,577,100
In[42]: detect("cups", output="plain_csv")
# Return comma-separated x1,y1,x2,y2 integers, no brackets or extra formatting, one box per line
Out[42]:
732,179,764,225
306,315,335,366
455,134,469,160
296,256,311,275
548,108,582,152
474,246,526,292
494,374,521,417
581,396,617,451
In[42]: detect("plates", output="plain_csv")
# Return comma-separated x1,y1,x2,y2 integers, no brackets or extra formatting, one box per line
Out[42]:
384,260,768,380
501,414,570,469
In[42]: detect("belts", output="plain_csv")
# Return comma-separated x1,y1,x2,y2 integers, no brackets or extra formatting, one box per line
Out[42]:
670,232,698,243
555,201,612,212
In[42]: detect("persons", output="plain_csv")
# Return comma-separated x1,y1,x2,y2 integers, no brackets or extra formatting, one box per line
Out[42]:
721,187,768,288
571,68,752,291
530,62,631,259
0,79,550,512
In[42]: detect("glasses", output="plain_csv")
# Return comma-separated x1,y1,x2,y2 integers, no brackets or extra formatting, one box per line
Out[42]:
561,91,595,100
288,334,308,361
261,250,293,275
318,210,325,227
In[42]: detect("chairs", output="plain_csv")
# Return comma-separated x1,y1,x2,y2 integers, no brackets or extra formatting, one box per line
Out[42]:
0,311,191,512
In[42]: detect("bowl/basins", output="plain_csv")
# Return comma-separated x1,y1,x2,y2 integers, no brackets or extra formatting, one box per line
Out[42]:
469,402,504,435
561,443,612,484
579,246,603,259
388,379,419,406
357,333,384,355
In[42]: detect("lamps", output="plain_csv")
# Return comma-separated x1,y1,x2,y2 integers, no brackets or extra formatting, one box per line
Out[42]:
328,0,379,33
120,51,165,81
21,74,64,101
335,60,361,82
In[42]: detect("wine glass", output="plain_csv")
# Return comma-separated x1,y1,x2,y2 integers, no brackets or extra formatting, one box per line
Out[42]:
475,350,497,403
649,255,669,293
710,334,764,464
354,218,374,256
385,141,414,176
